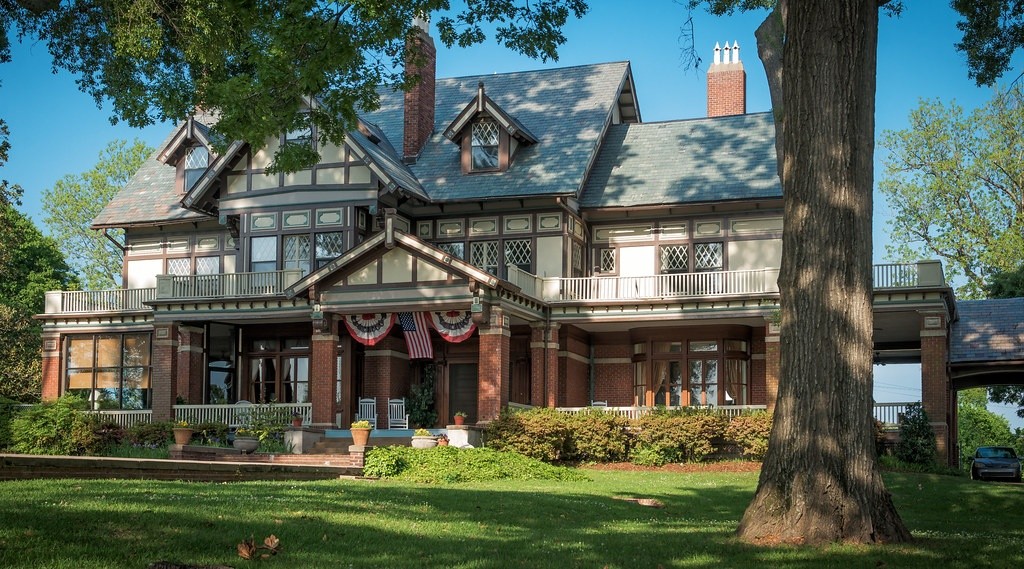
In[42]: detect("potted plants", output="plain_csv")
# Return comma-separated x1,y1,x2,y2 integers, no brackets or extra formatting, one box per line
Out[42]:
349,420,375,445
233,427,261,454
435,434,448,446
453,411,468,425
290,418,304,427
412,428,438,448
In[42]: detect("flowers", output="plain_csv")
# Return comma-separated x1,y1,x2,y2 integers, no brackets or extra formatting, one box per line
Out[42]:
177,420,190,428
292,411,302,418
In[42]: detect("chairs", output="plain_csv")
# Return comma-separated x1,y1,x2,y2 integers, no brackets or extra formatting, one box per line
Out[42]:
387,397,409,431
354,395,378,430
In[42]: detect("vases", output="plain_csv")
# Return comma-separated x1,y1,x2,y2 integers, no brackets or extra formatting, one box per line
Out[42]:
171,428,195,445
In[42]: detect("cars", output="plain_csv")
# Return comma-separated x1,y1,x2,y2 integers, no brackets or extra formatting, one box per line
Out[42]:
966,446,1023,482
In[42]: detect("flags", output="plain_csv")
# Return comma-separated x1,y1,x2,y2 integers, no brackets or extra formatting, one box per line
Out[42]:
397,311,434,361
